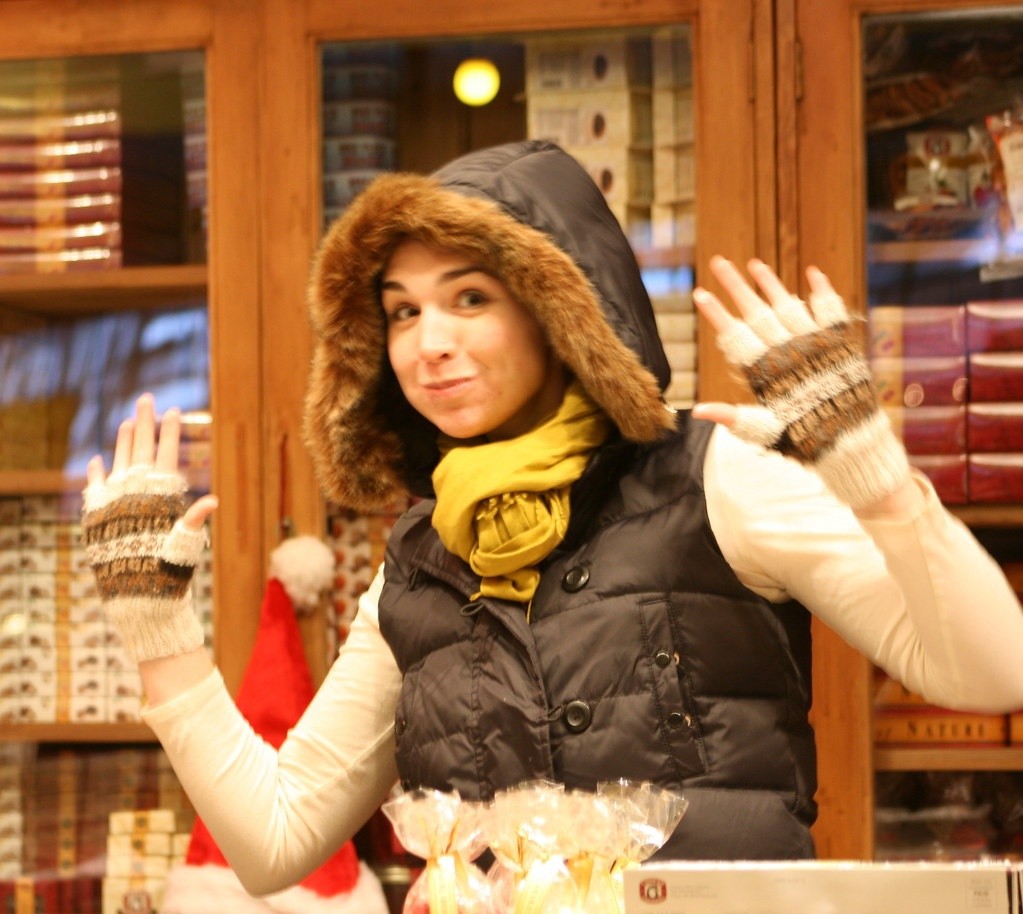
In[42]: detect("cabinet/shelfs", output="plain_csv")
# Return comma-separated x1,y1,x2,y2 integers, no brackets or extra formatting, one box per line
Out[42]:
0,0,1022,914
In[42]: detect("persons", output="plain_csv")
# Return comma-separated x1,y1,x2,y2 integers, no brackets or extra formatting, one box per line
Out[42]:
81,139,1023,899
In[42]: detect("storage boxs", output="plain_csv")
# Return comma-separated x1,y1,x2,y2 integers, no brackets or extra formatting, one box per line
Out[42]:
1,392,220,913
326,499,400,668
869,299,1023,505
519,25,698,411
